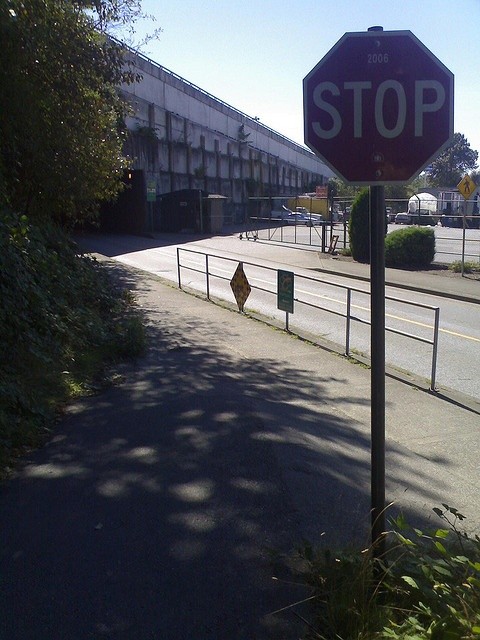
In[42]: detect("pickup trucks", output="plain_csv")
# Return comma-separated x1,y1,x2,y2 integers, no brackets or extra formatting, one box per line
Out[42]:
283,206,322,221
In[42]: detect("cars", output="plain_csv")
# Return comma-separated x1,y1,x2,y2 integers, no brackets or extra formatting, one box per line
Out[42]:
282,213,317,227
395,212,413,225
386,206,395,223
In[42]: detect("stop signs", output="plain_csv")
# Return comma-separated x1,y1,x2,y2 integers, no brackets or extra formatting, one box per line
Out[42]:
300,30,455,188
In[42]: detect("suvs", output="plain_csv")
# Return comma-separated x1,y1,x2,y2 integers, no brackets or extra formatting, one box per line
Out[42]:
343,206,352,221
414,209,438,225
